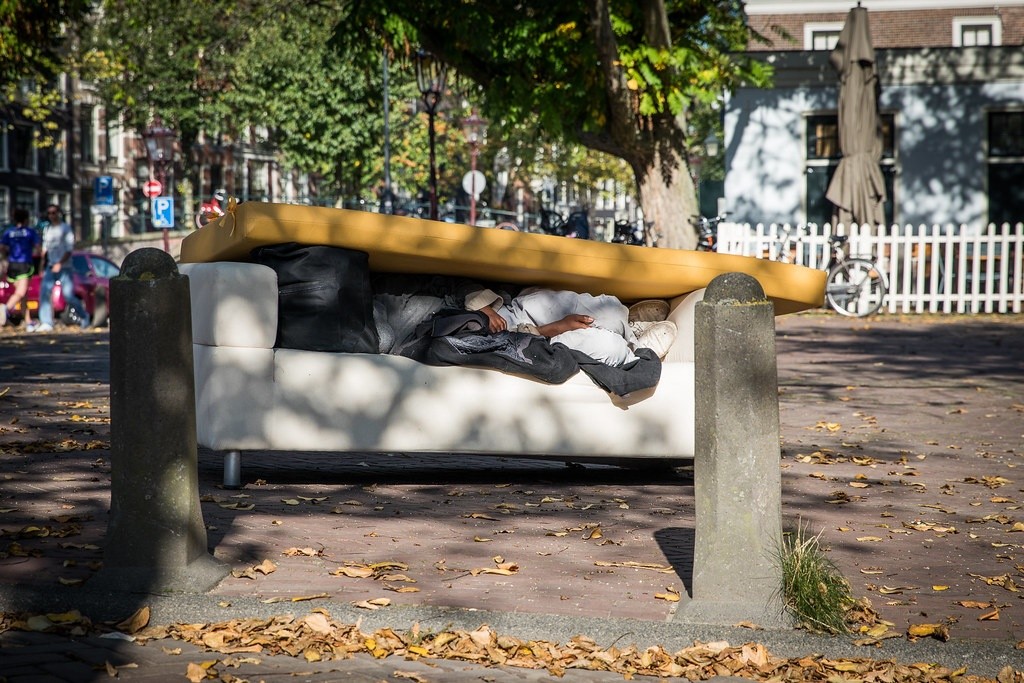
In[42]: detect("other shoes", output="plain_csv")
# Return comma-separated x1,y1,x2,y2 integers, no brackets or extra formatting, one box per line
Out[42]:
80,312,91,328
630,320,677,360
628,299,671,326
35,323,54,333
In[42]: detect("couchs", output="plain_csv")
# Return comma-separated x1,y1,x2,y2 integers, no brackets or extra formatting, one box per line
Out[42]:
179,259,706,487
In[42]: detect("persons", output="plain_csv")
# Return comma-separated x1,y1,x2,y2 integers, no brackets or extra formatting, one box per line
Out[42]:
371,274,670,397
35,205,90,332
0,209,41,332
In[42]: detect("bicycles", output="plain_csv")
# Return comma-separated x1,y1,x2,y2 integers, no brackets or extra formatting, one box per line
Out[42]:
613,211,728,253
772,223,888,318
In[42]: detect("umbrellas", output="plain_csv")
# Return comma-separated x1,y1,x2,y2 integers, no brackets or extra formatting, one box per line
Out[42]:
825,3,887,254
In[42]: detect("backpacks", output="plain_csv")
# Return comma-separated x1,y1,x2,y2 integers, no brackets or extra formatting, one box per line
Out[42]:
426,310,580,385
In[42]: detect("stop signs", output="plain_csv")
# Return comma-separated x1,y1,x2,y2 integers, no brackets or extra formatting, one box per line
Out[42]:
144,180,161,199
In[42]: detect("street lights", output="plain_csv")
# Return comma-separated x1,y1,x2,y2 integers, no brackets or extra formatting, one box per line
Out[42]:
461,108,491,227
414,49,450,221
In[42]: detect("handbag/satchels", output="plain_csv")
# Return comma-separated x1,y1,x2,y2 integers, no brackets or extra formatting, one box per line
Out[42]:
245,242,381,354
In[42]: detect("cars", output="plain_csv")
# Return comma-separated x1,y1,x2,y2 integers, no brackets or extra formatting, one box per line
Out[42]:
1,252,121,327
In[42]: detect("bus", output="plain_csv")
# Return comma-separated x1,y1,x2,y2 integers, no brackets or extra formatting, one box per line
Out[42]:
419,205,541,232
